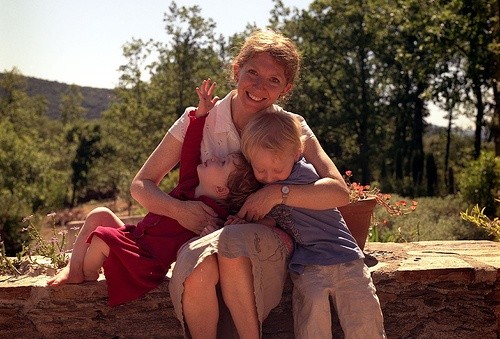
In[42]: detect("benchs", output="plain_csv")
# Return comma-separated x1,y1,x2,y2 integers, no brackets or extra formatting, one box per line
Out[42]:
0,237,499,338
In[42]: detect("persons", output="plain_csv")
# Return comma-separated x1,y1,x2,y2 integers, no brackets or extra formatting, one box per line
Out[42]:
130,26,352,338
43,77,258,305
222,109,389,338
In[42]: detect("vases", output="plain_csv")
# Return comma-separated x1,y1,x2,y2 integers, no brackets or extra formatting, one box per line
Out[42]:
337,197,378,250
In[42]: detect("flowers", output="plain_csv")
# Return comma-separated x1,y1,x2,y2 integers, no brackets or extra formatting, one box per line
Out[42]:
0,210,82,277
346,170,420,228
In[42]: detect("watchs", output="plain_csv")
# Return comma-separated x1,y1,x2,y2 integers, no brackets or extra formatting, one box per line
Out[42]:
280,182,291,205
